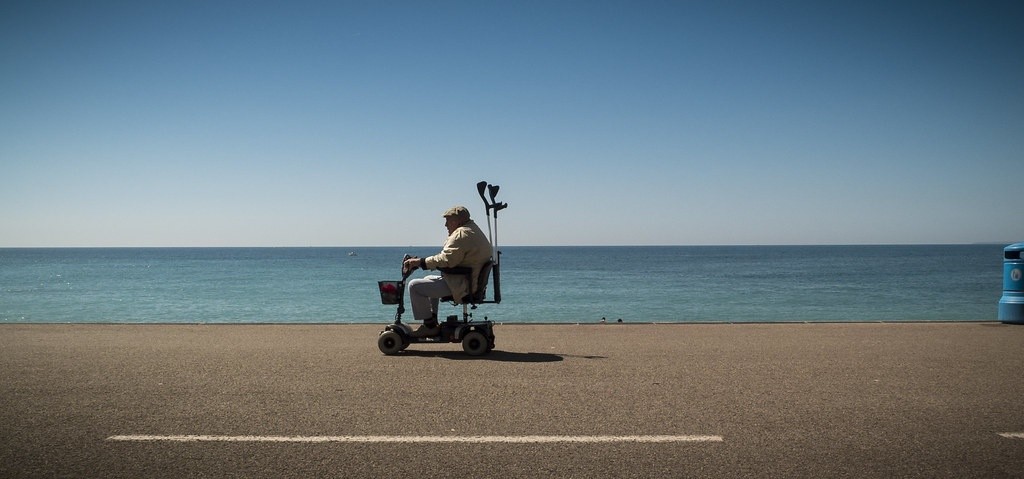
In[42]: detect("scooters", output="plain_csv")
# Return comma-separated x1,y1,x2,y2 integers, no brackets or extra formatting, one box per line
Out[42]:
377,180,507,355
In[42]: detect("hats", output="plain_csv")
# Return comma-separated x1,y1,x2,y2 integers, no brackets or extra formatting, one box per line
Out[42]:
443,206,470,218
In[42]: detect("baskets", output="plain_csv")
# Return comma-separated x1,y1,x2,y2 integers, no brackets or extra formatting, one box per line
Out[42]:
378,280,401,305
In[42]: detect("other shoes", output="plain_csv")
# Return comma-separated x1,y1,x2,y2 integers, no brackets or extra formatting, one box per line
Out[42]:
410,325,438,337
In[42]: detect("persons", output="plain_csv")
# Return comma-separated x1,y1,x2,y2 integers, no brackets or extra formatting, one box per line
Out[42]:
403,207,493,337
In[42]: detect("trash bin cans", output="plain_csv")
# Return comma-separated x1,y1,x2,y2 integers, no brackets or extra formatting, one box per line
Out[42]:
997,242,1024,325
